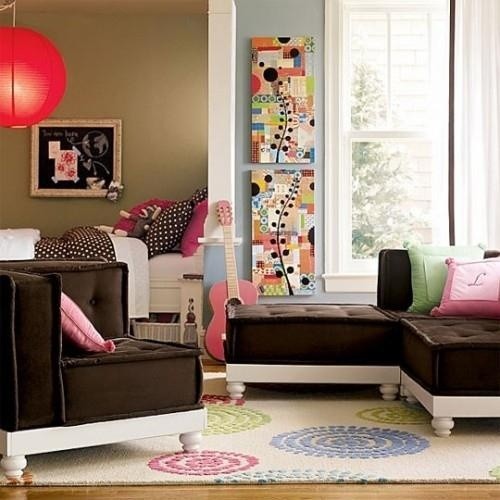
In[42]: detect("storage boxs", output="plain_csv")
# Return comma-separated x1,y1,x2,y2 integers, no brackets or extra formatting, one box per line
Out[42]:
131,317,200,344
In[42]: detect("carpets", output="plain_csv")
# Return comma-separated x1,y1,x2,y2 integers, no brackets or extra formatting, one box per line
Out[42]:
0,355,500,490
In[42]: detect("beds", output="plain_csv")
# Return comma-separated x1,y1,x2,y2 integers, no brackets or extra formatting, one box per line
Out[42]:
0,183,210,322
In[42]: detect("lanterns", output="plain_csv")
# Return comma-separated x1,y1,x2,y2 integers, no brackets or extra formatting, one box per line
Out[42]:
0,0,68,128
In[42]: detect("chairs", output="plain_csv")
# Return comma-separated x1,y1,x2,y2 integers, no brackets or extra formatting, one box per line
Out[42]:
1,252,207,479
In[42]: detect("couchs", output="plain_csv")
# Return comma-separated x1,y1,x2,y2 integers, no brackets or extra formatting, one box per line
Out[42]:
217,239,500,402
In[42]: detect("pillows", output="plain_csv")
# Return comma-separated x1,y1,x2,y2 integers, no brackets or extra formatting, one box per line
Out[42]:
114,198,174,238
192,186,207,204
401,237,491,314
181,199,208,260
59,289,116,355
428,253,500,319
144,192,195,259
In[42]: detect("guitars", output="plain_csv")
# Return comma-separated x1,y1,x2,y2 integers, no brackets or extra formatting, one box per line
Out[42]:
204,201,258,361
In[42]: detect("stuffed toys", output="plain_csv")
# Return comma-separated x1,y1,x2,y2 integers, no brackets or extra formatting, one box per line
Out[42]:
114,205,161,237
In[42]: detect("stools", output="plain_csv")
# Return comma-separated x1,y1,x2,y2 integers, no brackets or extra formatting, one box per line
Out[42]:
395,301,499,439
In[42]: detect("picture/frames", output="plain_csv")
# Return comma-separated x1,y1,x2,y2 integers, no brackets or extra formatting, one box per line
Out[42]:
29,116,125,201
